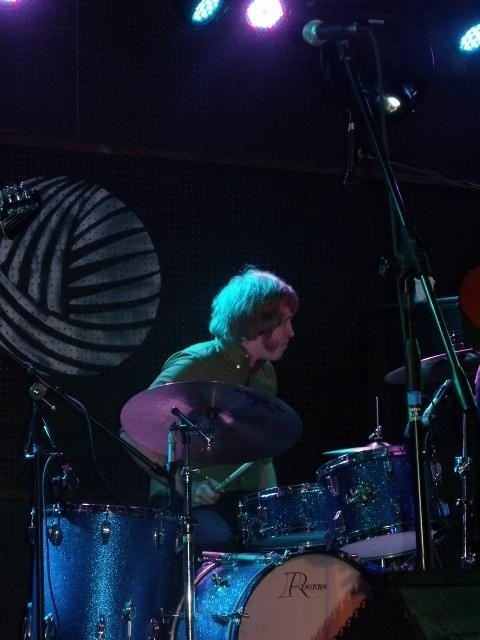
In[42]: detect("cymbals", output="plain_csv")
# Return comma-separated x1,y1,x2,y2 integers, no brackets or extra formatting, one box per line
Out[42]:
327,440,393,454
122,380,303,466
386,349,479,386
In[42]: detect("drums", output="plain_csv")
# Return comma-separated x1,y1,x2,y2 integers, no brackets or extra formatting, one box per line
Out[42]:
317,446,447,559
172,549,389,640
238,484,338,551
25,501,199,640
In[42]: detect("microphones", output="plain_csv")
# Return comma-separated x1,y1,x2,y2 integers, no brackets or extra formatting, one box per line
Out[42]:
301,19,359,42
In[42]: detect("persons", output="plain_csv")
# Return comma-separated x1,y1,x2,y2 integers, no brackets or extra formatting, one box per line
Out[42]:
120,268,299,555
